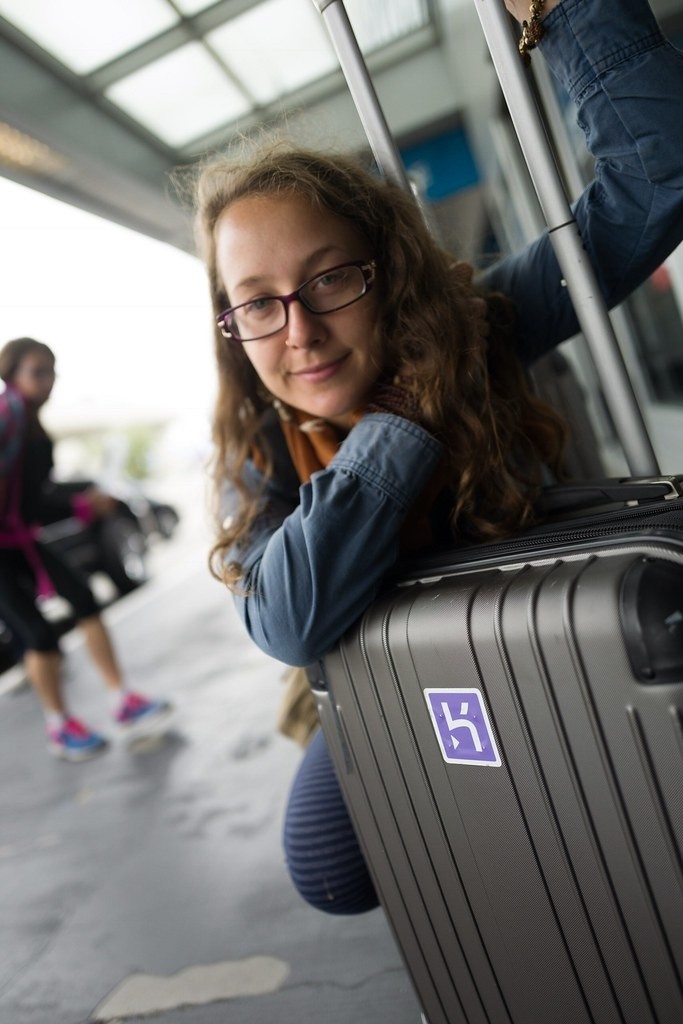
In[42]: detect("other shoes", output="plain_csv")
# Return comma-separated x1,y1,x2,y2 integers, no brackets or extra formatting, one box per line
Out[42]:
46,717,110,761
114,694,170,729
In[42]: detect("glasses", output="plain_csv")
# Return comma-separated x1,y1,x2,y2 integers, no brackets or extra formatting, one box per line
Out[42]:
217,249,377,341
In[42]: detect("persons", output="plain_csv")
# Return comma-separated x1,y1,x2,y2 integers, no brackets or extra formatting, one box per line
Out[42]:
189,0,683,915
1,338,174,761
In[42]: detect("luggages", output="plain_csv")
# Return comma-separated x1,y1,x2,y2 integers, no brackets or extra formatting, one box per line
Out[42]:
305,0,683,1024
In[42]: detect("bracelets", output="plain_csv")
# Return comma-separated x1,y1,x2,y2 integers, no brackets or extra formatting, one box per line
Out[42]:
519,0,547,54
